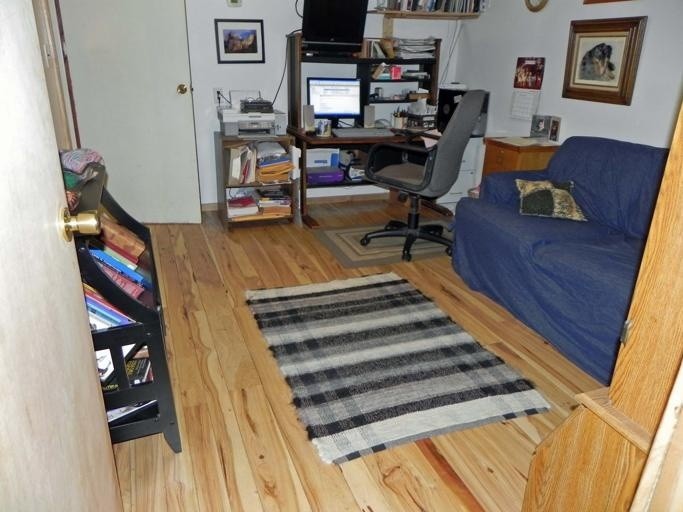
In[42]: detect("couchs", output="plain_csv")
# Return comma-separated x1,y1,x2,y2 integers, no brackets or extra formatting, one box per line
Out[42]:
451,135,670,387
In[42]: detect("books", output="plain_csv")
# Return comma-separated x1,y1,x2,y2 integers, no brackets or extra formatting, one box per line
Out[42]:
226,141,293,223
393,0,481,14
81,209,158,427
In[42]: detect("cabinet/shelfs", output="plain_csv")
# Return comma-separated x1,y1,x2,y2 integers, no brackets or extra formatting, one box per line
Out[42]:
287,30,455,231
55,145,181,456
434,137,486,206
477,136,558,183
213,129,298,233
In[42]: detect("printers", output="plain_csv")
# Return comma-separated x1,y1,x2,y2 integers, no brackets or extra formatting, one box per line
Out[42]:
216,105,288,139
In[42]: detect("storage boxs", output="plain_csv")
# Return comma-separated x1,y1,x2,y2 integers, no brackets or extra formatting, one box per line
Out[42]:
305,148,368,186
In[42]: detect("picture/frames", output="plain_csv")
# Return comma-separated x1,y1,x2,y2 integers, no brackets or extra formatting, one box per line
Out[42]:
212,17,266,65
560,14,649,106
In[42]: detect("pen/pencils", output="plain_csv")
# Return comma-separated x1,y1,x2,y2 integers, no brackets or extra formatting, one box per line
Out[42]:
393,106,408,117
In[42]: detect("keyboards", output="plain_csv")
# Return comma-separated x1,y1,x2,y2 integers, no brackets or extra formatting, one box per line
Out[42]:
332,128,396,138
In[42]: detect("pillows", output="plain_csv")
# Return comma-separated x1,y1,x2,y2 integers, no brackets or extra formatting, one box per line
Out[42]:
514,176,590,224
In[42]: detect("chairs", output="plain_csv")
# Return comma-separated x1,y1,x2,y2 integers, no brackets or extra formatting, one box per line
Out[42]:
359,88,488,262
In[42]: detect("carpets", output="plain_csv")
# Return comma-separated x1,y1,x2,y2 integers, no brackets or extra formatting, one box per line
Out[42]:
242,271,552,469
312,218,452,270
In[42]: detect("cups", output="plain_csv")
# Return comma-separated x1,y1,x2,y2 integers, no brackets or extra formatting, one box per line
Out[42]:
374,88,382,98
317,119,332,136
390,114,408,129
377,1,384,10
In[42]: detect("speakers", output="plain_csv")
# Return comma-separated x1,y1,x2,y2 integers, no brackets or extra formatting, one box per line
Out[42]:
363,104,376,127
302,104,315,130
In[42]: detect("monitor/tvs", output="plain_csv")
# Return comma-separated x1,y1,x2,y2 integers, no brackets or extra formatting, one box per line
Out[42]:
306,77,362,127
301,0,368,46
